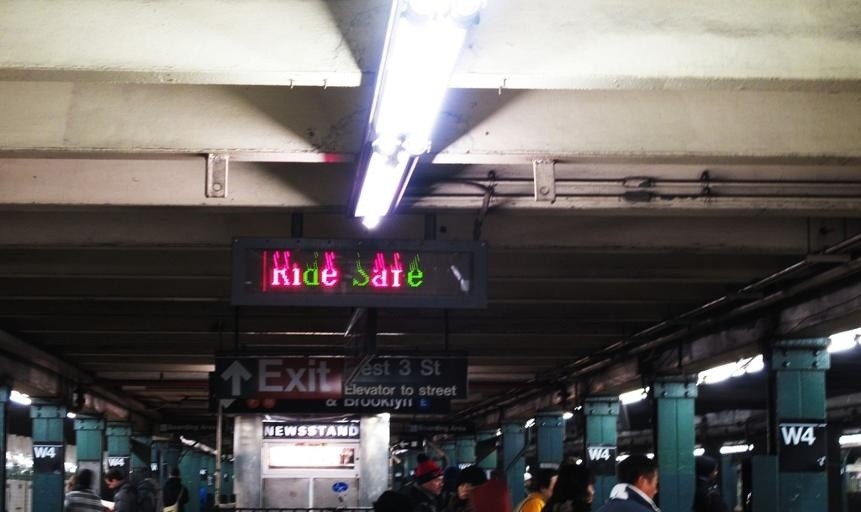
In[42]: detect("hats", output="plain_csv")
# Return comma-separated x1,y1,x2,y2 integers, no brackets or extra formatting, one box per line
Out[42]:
417,454,444,485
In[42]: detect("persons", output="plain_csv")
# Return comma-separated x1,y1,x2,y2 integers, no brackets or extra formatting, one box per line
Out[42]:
593,454,661,512
541,457,597,510
512,465,559,510
65,465,237,512
374,452,512,511
694,457,743,512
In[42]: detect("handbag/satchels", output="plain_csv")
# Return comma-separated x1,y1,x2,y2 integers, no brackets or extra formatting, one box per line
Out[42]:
163,503,178,512
